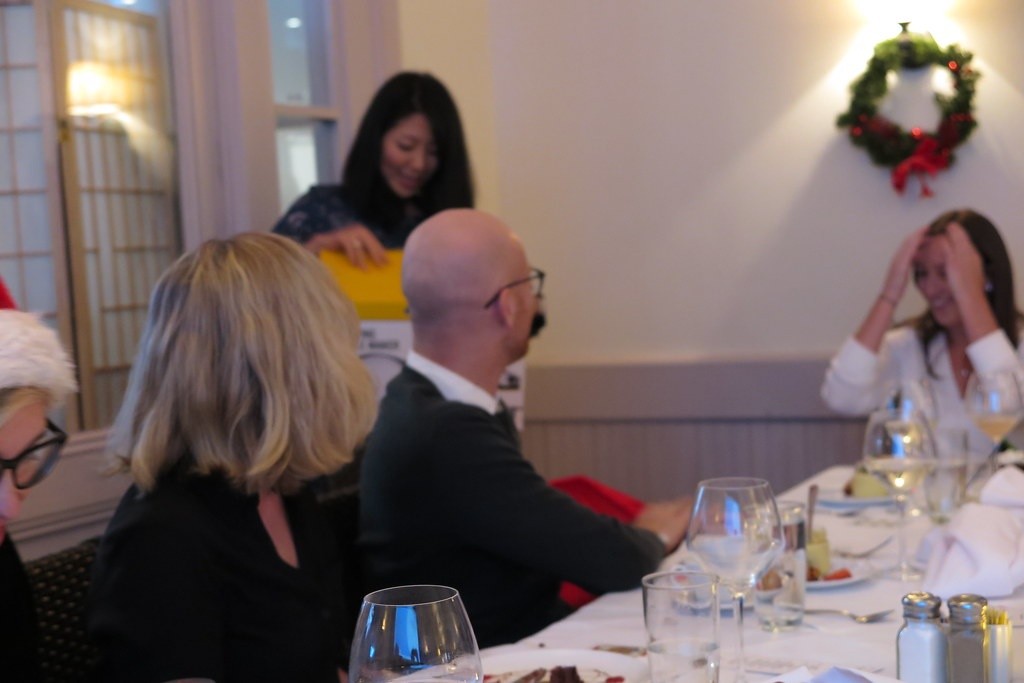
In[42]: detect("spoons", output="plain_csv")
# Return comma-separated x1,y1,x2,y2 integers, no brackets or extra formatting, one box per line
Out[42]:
782,606,894,624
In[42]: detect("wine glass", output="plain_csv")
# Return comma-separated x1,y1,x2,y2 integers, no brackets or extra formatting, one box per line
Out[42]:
862,408,936,581
965,367,1024,476
686,476,785,683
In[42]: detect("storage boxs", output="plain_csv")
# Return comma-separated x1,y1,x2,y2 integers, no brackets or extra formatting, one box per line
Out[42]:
312,244,413,325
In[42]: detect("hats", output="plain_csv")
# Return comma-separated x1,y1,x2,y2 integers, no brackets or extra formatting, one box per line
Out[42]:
0,276,79,405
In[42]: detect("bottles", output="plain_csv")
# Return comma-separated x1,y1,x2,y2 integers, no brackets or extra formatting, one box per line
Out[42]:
895,589,949,683
944,593,988,683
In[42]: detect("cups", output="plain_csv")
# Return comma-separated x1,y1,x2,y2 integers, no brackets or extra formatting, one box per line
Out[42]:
645,637,719,683
347,584,484,683
923,428,969,526
748,499,808,635
640,570,721,654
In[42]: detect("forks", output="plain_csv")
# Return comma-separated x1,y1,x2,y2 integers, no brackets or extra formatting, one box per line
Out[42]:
832,535,894,560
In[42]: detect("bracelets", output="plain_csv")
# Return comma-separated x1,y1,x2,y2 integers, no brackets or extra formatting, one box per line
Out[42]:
880,293,898,305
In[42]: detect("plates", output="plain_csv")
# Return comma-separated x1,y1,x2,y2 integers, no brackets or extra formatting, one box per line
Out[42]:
423,647,650,683
805,555,870,591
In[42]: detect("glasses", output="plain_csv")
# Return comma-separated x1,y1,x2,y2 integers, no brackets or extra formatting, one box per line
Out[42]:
0,417,68,489
482,268,544,310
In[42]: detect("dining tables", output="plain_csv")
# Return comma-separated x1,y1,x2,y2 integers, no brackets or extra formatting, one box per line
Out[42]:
477,447,1024,682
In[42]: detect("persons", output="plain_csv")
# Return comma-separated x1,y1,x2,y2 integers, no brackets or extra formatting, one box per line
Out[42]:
356,208,702,649
266,74,476,269
0,307,215,683
821,209,1024,470
89,231,406,683
